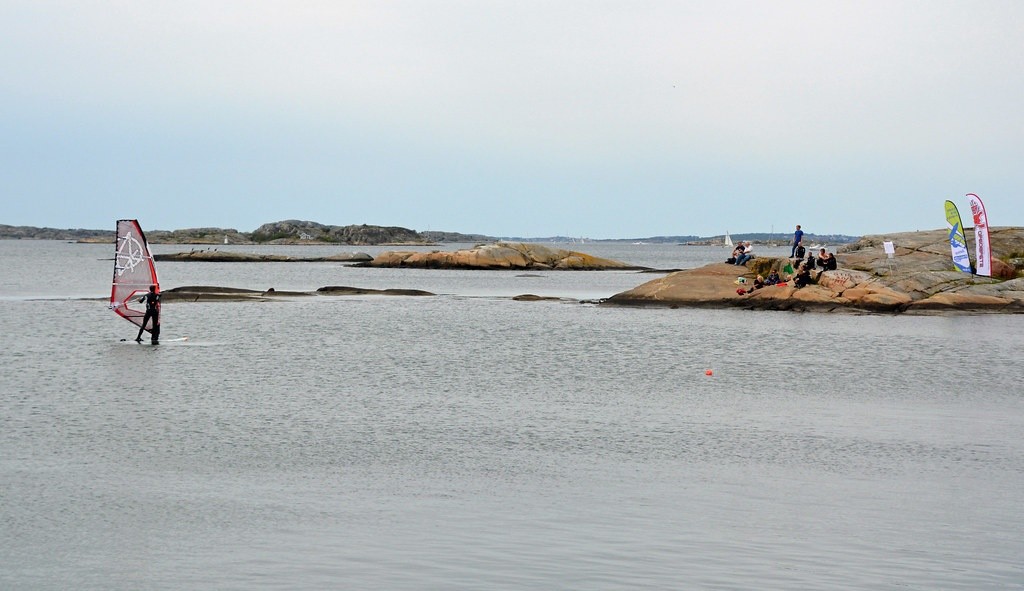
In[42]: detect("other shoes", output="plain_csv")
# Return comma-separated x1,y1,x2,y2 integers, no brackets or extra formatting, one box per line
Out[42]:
733,264,737,266
789,256,794,258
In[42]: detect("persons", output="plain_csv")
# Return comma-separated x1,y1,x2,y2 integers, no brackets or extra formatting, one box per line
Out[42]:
793,268,806,289
754,269,779,289
789,225,805,269
817,249,837,271
732,241,754,266
134,284,161,345
803,251,815,271
